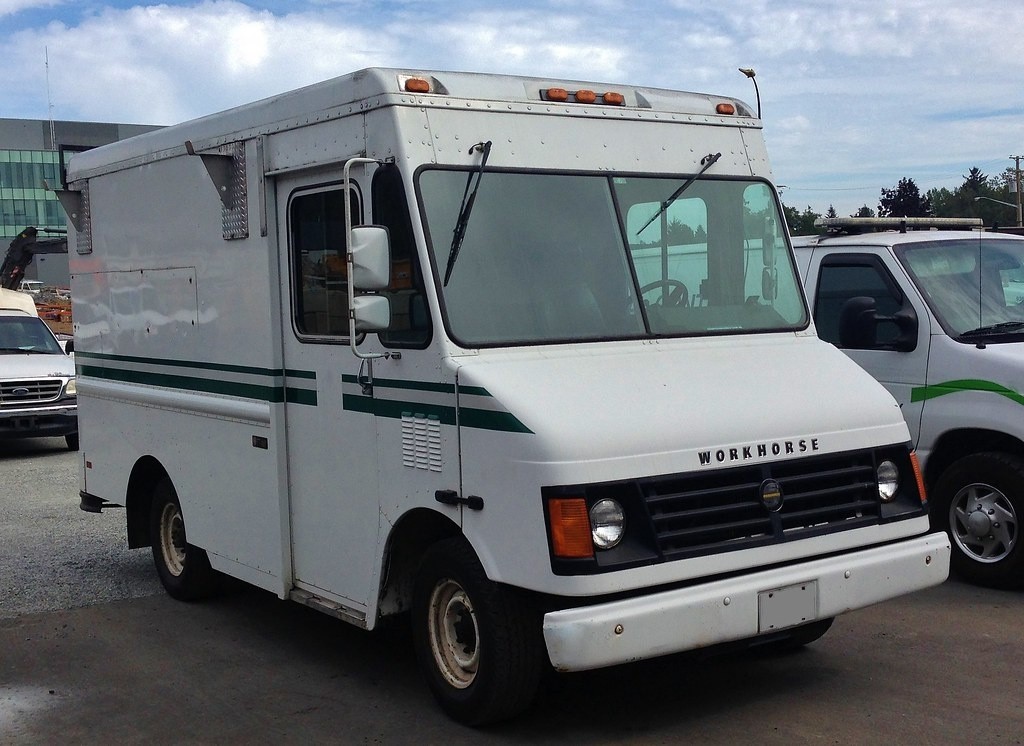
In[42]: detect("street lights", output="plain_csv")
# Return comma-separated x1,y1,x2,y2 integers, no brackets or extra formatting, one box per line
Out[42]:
738,66,762,120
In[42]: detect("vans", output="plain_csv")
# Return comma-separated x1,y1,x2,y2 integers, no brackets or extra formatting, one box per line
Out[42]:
0,287,79,451
627,216,1024,584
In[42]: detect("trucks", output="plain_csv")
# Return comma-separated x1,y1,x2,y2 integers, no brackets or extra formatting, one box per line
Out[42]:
56,67,951,726
